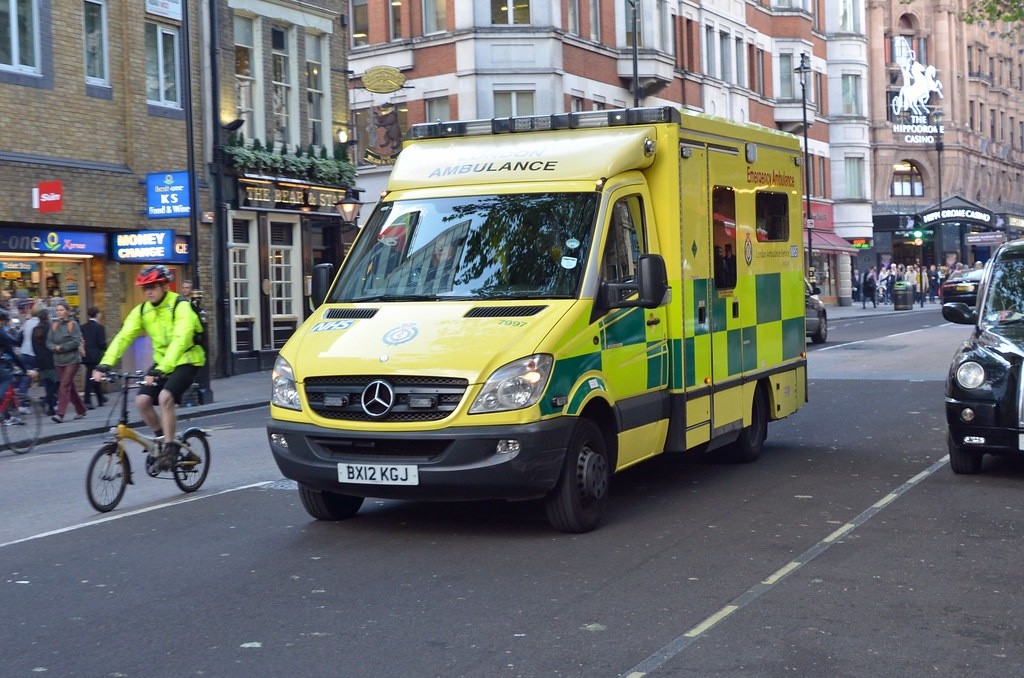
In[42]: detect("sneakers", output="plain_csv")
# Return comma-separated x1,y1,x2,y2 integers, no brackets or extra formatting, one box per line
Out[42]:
3,416,23,426
153,447,179,471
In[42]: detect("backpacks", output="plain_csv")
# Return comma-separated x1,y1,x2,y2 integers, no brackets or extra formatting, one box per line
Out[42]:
53,320,86,358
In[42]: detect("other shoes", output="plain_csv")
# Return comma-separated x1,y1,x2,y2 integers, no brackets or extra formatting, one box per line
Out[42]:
99,398,108,406
18,407,31,415
74,411,87,419
51,415,64,423
85,403,95,410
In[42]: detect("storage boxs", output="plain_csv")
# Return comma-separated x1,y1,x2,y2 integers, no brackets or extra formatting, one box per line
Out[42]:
0,291,12,301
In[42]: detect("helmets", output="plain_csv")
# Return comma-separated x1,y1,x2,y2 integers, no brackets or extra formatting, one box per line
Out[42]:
135,264,173,285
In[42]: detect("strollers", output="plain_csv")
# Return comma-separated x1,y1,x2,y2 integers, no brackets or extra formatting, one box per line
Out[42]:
875,282,888,306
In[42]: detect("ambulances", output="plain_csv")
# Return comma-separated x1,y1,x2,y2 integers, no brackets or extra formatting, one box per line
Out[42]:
267,105,809,533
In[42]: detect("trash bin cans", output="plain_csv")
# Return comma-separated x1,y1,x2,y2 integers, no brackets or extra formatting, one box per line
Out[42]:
894,282,913,310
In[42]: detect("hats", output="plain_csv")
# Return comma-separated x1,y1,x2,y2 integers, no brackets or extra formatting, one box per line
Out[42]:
58,301,70,313
955,262,964,266
38,307,53,319
976,261,982,265
0,310,11,320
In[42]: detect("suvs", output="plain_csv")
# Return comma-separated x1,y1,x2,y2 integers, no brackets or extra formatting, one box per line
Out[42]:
941,232,1024,474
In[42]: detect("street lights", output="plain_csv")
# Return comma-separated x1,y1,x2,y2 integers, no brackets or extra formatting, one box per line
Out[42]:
929,107,946,264
794,53,821,295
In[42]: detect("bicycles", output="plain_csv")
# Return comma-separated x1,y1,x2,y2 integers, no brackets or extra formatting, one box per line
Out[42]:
85,370,210,512
0,369,41,454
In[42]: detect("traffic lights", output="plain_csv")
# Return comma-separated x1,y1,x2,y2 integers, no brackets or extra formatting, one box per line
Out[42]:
914,214,922,246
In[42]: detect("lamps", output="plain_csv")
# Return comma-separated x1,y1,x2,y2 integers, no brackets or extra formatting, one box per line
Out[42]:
223,118,246,132
332,186,364,226
340,138,358,149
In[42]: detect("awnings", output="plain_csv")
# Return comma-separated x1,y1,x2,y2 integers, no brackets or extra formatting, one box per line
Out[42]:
803,230,860,256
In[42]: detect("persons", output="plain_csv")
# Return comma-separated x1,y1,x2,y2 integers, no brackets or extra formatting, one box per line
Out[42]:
181,279,207,391
92,266,207,477
0,290,57,424
852,269,861,302
45,302,87,423
80,307,109,409
405,236,455,289
863,261,983,309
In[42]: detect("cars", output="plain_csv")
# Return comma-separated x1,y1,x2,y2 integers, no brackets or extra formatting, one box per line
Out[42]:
941,269,984,306
805,275,827,344
